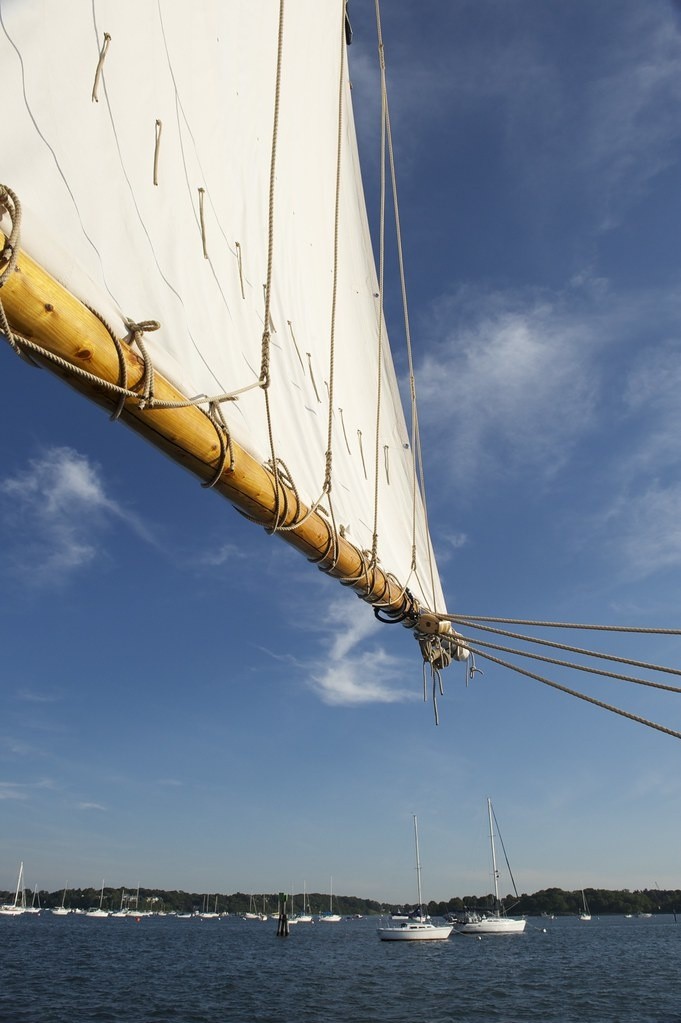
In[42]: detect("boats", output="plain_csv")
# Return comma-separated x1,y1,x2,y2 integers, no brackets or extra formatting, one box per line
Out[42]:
636,913,652,918
625,914,632,918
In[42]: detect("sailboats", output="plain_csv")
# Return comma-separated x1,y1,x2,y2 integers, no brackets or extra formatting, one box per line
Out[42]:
377,814,454,940
579,888,591,921
0,861,342,924
456,797,527,934
